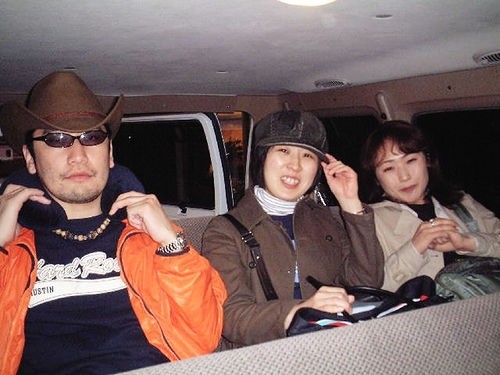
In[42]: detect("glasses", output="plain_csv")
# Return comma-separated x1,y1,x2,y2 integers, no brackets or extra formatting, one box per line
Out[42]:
33,131,110,148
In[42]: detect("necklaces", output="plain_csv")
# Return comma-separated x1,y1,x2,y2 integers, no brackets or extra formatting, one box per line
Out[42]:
49,217,113,242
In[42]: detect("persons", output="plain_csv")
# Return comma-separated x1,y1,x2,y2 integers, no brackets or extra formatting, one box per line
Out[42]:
0,71,229,375
359,119,500,294
199,109,385,347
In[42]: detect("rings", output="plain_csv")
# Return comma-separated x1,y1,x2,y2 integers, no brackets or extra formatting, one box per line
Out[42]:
429,219,435,227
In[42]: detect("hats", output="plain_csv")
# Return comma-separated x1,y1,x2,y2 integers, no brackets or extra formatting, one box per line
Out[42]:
0,71,125,145
254,109,331,165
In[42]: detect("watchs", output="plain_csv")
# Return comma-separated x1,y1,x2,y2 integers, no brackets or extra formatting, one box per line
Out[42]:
155,232,187,255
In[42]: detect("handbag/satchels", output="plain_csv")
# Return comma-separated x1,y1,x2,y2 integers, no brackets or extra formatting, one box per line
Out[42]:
287,274,446,337
434,255,500,301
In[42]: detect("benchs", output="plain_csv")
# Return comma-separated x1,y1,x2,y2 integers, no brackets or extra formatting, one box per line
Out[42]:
117,216,500,375
